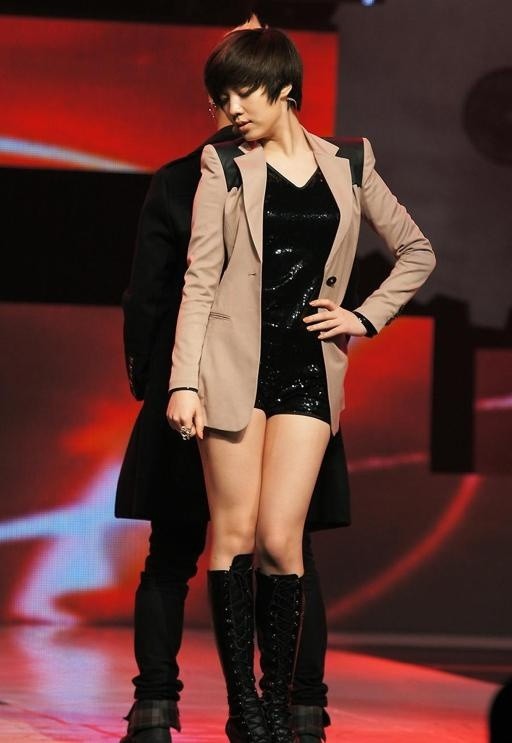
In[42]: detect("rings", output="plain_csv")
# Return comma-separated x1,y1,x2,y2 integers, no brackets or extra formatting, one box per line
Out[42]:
180,424,194,440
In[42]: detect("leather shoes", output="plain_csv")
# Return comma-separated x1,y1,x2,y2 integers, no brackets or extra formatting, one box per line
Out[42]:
289,705,331,743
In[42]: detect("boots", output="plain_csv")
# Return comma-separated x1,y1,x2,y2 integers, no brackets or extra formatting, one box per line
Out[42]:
119,699,180,742
207,552,307,743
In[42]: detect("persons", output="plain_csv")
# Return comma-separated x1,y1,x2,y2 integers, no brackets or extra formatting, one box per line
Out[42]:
166,28,436,743
114,13,351,743
